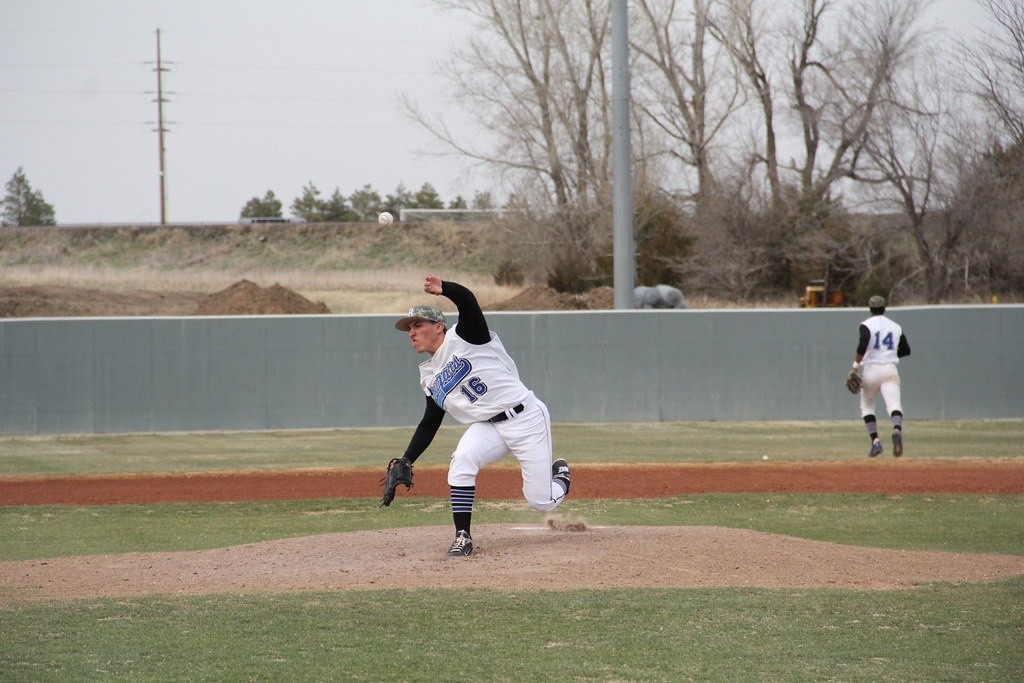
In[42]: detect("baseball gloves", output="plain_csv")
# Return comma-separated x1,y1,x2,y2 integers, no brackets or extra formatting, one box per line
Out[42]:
846,373,863,394
382,456,414,507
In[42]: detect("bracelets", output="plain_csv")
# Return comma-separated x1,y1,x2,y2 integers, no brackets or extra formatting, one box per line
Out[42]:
853,362,859,369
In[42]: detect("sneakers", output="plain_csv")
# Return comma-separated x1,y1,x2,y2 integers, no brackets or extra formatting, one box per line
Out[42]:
446,530,473,557
552,458,571,482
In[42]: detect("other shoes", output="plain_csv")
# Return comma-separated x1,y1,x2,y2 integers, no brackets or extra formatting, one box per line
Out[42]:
891,429,903,457
868,441,882,458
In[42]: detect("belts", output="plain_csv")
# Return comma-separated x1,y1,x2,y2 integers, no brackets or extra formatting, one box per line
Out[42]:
487,404,524,422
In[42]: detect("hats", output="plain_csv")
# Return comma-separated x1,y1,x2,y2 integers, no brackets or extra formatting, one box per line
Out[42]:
395,305,446,332
868,296,886,307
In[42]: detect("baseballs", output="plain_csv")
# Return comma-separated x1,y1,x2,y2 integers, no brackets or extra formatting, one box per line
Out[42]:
761,454,769,461
377,212,394,228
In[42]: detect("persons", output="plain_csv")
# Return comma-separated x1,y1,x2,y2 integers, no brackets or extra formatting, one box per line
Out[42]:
378,274,571,556
845,295,911,457
